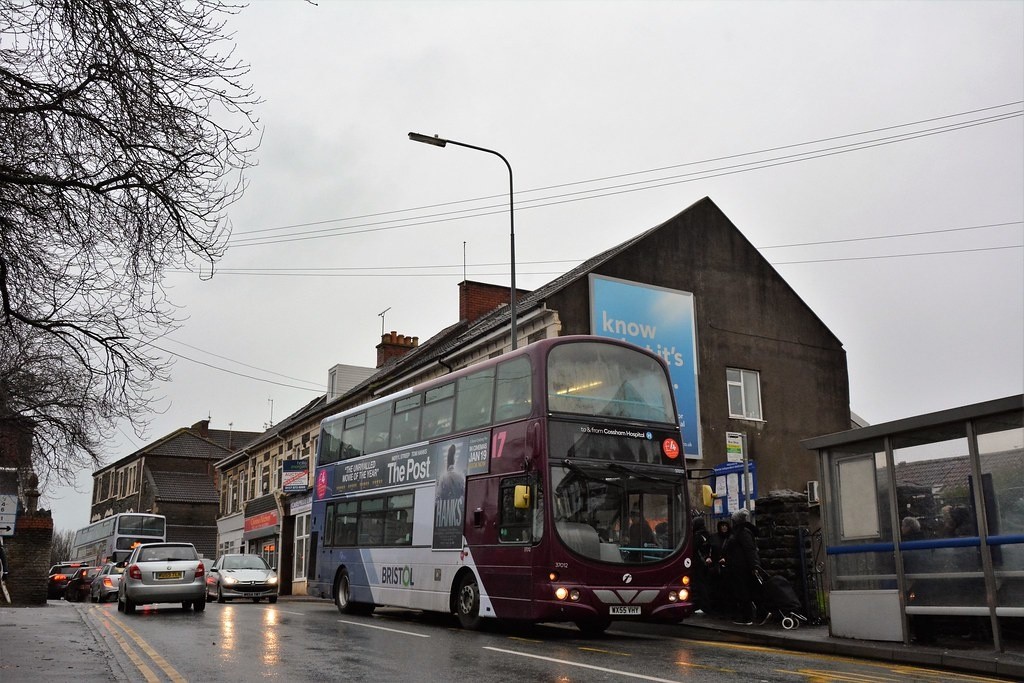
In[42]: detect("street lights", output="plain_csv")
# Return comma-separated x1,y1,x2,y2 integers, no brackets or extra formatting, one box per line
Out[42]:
406,133,518,354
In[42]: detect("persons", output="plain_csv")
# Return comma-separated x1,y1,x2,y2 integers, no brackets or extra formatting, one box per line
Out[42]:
434,445,466,505
892,504,985,644
621,502,772,626
336,409,444,545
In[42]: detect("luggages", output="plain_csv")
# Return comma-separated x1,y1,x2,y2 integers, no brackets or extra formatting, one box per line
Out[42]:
755,569,808,629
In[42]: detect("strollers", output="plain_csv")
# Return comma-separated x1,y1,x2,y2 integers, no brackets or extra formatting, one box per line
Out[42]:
752,565,807,630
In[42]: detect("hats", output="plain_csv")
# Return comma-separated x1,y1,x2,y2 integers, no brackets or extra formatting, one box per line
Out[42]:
733,512,747,525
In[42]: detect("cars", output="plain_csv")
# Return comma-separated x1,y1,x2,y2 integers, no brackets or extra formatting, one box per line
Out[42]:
205,554,278,604
117,542,205,614
48,562,127,603
200,559,215,578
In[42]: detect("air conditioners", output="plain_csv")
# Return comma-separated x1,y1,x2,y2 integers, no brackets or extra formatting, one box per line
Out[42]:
807,481,819,503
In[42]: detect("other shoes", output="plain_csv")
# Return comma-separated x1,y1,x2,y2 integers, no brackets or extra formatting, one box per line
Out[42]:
758,611,772,626
695,609,706,614
731,617,754,626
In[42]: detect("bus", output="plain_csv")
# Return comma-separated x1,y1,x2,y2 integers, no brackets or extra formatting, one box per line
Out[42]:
67,513,166,566
304,334,692,640
